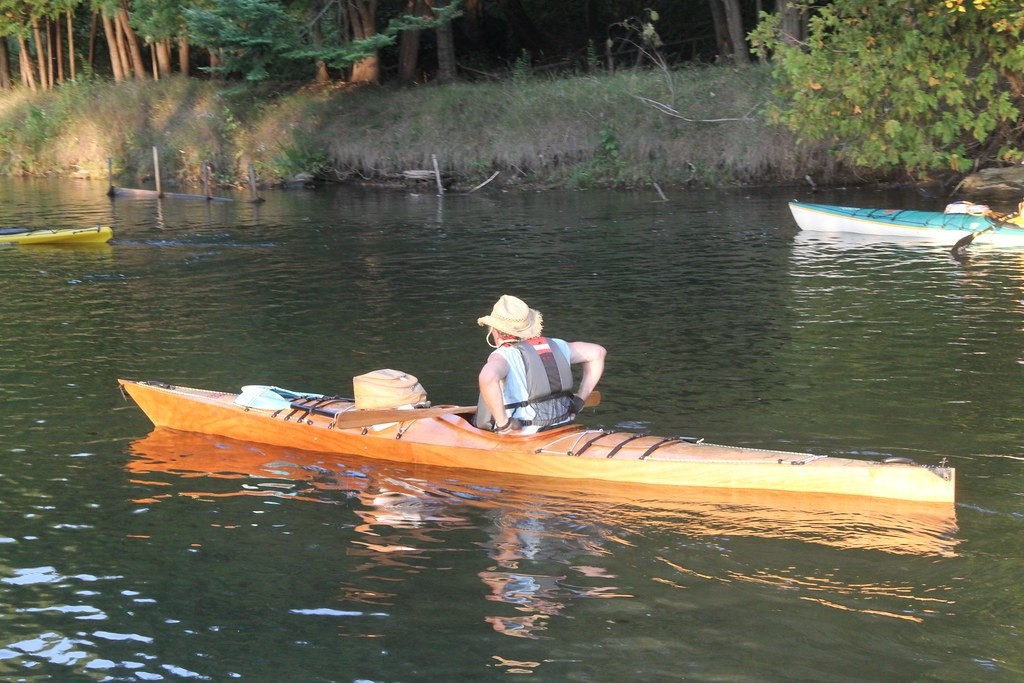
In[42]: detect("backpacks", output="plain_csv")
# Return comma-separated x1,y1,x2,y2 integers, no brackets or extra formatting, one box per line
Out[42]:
353,368,428,410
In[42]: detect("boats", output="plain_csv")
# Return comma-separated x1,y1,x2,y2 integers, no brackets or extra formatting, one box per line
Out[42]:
0,224,115,246
787,199,1023,248
117,377,956,510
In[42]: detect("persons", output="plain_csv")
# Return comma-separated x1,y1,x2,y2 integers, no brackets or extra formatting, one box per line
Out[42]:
471,295,607,437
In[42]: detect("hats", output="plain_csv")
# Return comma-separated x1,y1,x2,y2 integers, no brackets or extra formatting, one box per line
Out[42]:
477,295,544,340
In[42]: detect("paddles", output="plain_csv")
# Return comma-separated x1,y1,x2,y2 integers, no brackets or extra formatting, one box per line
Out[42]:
336,390,600,430
950,212,1018,258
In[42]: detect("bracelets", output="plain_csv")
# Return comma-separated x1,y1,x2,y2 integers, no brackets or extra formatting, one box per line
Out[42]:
495,418,510,430
